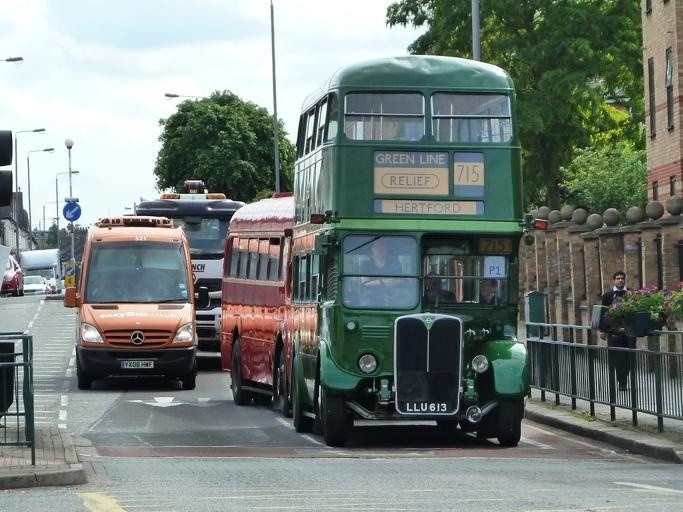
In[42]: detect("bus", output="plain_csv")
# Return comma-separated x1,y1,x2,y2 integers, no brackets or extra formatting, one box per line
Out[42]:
285,53,534,450
219,190,301,415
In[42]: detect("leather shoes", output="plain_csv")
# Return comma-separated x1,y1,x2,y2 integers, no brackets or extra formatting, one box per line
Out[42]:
618,380,627,391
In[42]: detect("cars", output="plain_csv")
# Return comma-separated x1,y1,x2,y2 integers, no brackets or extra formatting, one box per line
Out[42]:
0,254,25,297
22,275,48,295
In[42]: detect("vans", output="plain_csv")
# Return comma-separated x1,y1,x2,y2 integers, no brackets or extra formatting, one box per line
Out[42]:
64,213,210,392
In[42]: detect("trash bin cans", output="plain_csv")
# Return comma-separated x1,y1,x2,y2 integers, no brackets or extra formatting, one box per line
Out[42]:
64,287,76,307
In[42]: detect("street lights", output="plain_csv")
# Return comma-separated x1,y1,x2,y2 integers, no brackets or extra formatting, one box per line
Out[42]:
56,170,80,225
65,139,76,259
26,147,55,250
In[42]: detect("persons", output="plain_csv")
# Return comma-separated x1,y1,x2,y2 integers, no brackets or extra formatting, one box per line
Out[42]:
426,272,502,307
600,271,639,391
355,230,406,291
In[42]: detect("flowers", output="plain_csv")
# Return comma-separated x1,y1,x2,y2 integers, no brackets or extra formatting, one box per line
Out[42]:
602,279,682,333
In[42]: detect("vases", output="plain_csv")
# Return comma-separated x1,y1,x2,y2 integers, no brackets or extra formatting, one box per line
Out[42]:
623,308,662,338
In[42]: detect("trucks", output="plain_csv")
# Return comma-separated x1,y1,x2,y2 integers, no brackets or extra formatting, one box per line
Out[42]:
22,249,62,294
134,178,248,358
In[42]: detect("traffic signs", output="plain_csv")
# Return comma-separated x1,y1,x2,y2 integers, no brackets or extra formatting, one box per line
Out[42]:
15,128,47,192
